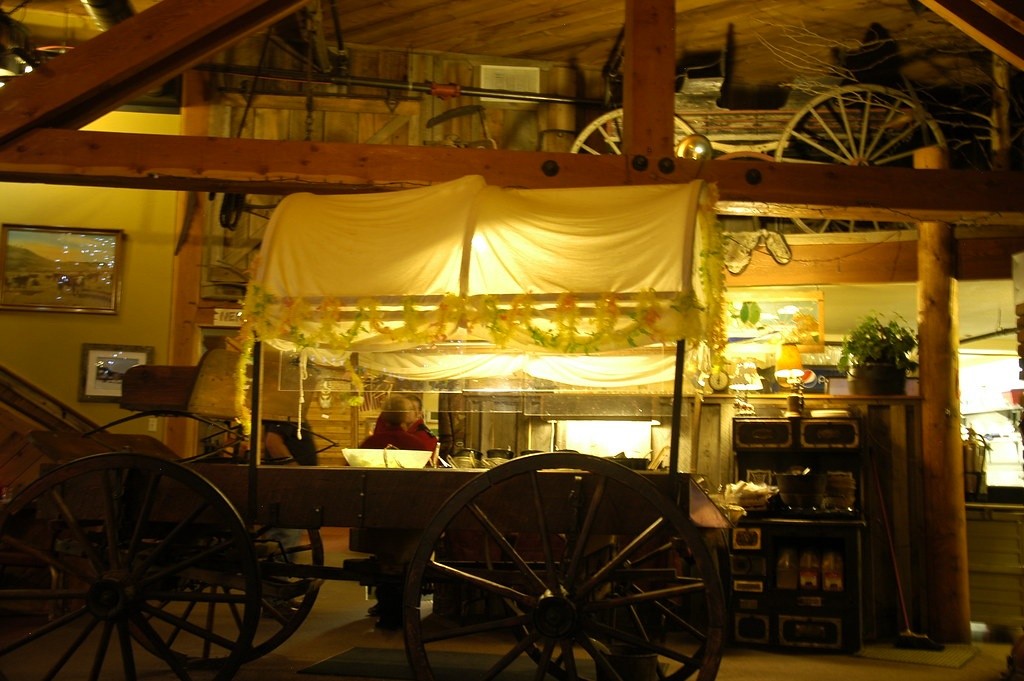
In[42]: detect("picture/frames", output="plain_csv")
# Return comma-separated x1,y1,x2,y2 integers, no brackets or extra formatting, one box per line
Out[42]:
77,343,156,402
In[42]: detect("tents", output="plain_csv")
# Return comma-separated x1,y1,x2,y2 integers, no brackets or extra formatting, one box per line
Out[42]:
231,176,727,472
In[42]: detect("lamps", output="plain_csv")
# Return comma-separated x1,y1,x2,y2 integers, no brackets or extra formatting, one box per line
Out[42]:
728,360,763,415
775,341,805,385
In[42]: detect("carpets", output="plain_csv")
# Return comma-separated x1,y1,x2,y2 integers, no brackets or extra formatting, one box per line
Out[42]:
856,642,979,668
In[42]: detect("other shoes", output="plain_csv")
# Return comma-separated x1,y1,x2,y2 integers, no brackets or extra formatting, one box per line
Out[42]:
262,602,295,618
284,599,302,607
376,615,403,631
369,602,382,616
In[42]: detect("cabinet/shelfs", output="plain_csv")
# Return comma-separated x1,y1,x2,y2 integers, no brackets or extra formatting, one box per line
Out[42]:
725,416,867,653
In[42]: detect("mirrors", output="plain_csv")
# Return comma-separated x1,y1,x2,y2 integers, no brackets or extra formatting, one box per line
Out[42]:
0,222,126,315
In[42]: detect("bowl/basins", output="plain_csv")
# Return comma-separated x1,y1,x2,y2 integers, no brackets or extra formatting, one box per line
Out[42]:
342,449,433,468
457,447,483,461
520,450,543,456
487,447,515,461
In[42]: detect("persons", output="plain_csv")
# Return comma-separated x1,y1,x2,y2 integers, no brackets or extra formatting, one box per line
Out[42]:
220,370,319,618
356,393,439,631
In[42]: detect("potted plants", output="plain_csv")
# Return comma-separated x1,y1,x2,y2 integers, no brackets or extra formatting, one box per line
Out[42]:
838,312,924,397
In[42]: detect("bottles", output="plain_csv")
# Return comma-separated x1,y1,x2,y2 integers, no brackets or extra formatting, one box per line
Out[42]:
776,549,842,591
